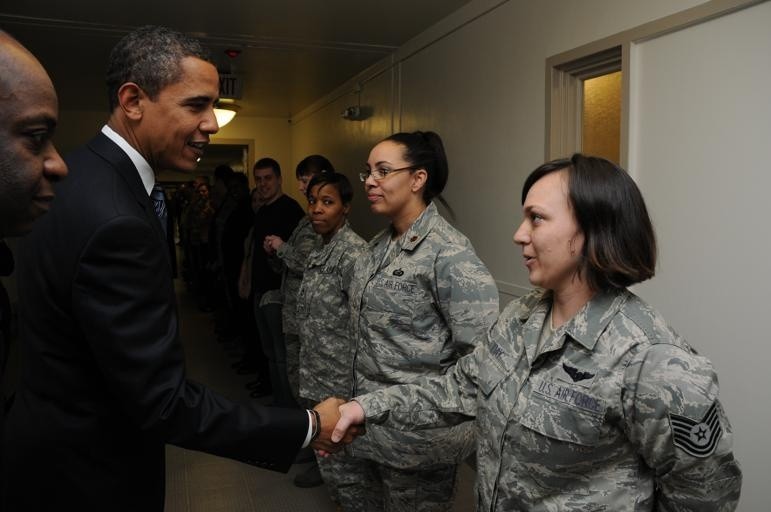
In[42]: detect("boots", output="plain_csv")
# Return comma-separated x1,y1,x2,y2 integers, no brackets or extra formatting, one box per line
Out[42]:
294,457,325,489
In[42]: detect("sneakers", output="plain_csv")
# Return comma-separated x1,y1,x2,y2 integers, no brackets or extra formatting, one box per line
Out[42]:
197,303,273,398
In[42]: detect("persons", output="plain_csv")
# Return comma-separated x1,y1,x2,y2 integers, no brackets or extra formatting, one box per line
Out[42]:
154,129,500,512
316,153,744,510
2,30,74,414
2,23,366,512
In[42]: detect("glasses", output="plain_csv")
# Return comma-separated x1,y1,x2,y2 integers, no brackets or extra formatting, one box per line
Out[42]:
359,165,417,182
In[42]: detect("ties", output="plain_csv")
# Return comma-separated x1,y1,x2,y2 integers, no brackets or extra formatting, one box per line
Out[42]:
154,186,170,237
